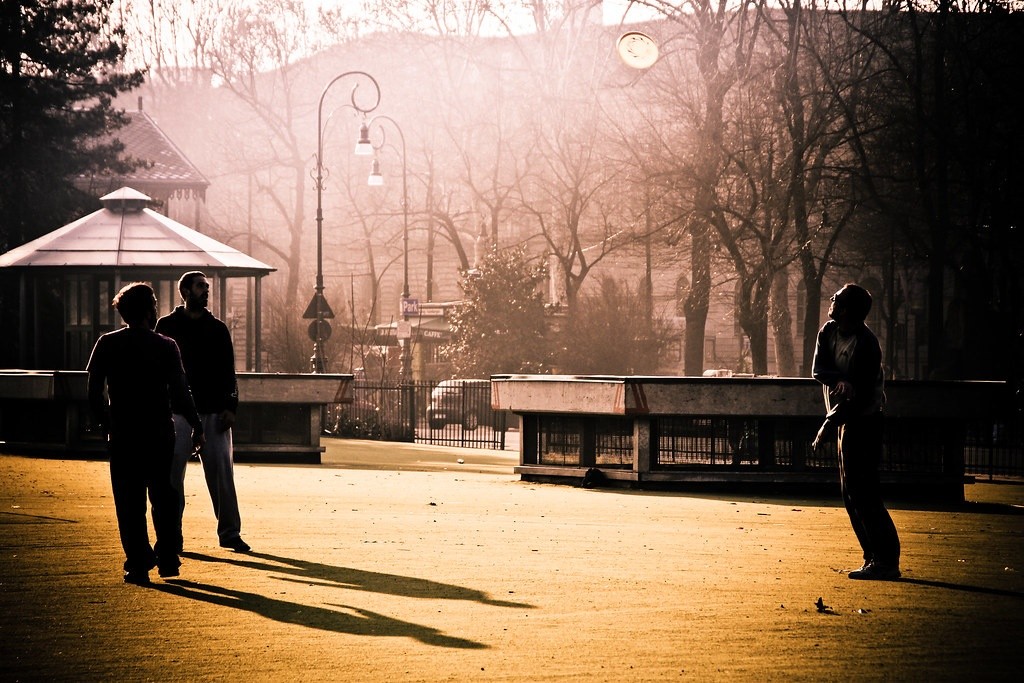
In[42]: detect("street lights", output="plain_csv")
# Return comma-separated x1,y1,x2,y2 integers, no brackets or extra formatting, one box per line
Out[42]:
367,115,415,425
310,69,381,375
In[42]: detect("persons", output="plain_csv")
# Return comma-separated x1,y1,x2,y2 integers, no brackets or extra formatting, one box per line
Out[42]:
810,285,903,580
155,272,250,553
87,284,204,585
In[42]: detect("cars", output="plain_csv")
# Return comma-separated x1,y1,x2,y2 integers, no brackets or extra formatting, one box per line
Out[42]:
427,380,517,431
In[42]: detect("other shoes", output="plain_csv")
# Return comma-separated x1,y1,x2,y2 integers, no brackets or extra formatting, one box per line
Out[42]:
158,564,179,577
219,536,250,552
848,559,901,581
125,572,149,584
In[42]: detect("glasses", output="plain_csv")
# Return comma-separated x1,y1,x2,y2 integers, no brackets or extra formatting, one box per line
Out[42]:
833,293,847,301
